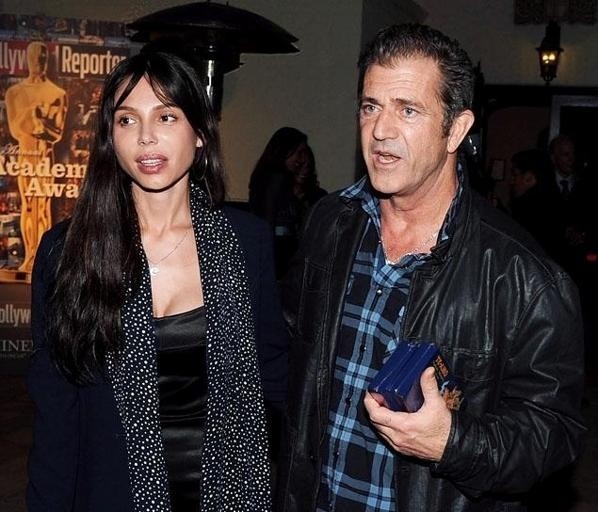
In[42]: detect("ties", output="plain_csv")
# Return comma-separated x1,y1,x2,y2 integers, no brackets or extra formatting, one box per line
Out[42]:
560,179,570,201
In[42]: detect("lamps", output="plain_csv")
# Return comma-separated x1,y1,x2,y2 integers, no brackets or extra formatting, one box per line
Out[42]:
535,0,564,85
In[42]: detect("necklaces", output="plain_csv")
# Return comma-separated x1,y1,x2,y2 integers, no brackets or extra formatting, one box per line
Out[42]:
382,231,440,263
138,227,193,276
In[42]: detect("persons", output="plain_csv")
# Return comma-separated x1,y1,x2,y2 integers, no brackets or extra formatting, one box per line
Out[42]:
4,41,68,272
248,126,329,224
280,24,597,512
25,51,287,512
513,136,598,278
